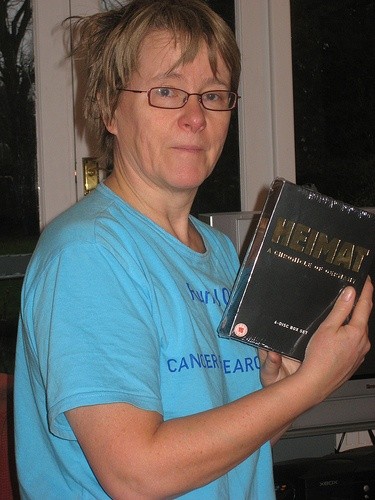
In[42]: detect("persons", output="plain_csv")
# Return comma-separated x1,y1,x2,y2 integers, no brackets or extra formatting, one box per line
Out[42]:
12,0,374,500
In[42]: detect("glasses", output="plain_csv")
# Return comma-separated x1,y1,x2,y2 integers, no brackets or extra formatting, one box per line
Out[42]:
115,86,242,111
